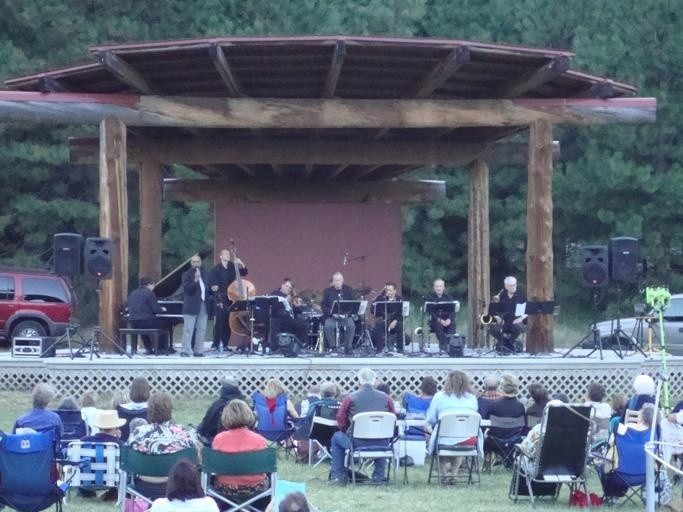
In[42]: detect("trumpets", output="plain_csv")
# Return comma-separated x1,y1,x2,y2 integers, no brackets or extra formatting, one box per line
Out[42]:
286,288,302,307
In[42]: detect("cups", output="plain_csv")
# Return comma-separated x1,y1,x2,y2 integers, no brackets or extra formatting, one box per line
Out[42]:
265,345,271,353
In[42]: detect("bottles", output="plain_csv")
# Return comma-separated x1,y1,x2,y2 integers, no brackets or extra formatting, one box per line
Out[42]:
219,339,223,355
258,344,263,352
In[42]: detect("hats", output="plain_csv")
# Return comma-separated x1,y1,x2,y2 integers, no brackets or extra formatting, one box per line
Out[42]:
93,411,126,430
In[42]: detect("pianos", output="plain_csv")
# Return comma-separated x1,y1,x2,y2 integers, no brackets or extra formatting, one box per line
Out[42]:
119,250,215,354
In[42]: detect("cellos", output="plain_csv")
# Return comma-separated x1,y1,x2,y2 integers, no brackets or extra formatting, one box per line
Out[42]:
227,247,257,336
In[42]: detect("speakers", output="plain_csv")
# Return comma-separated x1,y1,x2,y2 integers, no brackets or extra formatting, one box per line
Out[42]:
445,334,467,356
84,238,113,279
609,237,638,281
582,246,608,286
54,233,81,275
277,331,303,358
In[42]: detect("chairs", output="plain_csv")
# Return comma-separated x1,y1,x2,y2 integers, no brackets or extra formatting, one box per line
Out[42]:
2,407,197,512
253,391,523,487
201,447,277,512
505,393,681,510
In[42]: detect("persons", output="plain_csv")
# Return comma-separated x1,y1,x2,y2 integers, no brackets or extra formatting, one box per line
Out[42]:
320,271,356,356
425,278,456,351
370,281,411,357
489,276,527,356
179,255,219,357
207,248,249,352
270,277,299,353
127,274,177,355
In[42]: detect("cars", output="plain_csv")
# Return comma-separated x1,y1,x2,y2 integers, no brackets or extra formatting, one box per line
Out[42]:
591,292,683,358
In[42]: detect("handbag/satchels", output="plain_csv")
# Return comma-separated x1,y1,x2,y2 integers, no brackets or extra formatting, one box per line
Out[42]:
571,489,600,507
329,466,367,484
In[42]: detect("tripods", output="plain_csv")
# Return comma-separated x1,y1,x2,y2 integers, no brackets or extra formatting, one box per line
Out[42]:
562,291,648,358
40,290,133,360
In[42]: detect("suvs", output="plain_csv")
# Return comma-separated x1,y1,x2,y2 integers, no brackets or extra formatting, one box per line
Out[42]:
0,271,75,347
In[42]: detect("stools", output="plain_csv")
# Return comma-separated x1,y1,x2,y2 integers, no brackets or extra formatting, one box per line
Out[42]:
115,325,169,358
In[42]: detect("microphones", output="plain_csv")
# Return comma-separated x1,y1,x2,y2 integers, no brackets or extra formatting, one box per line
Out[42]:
342,250,348,266
230,238,234,253
